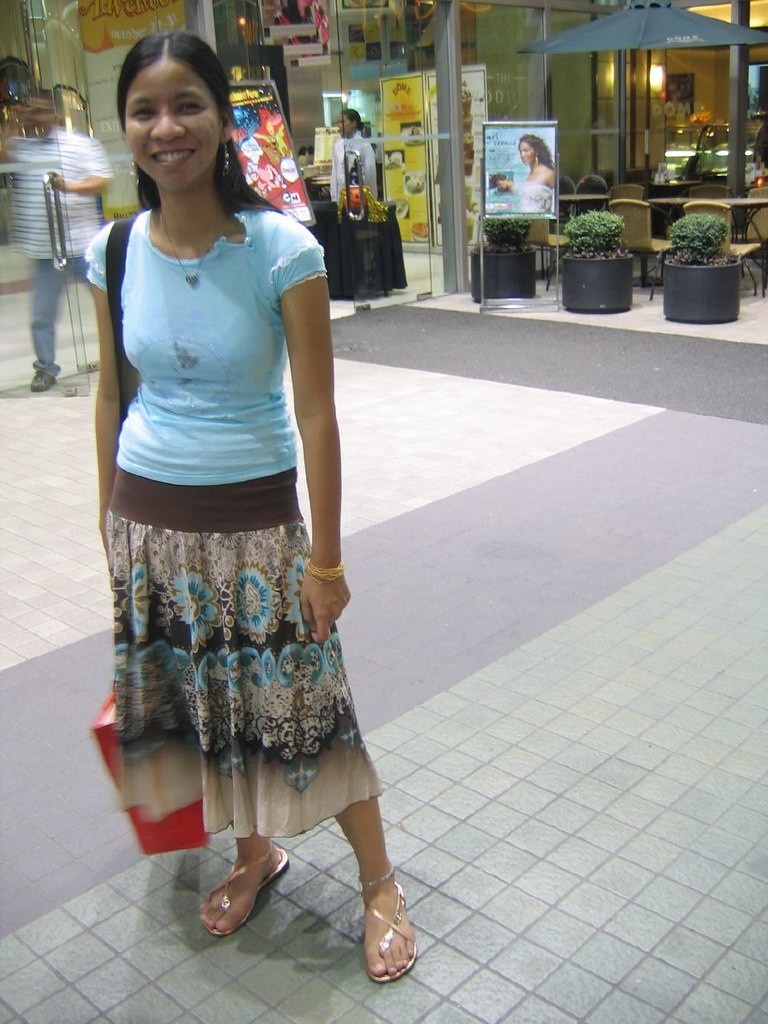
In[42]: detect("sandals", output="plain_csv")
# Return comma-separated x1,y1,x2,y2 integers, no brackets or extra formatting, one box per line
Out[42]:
204,847,291,935
356,872,418,982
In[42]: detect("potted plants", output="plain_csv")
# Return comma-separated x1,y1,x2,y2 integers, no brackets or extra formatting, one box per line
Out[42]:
469,215,538,304
660,212,742,324
559,209,635,314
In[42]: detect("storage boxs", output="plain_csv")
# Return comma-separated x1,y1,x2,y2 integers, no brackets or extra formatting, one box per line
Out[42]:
663,120,768,190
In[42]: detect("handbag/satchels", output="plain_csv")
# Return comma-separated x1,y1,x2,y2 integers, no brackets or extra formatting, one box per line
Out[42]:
94,688,212,854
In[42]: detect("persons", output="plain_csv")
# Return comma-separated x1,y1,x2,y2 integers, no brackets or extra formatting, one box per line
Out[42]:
519,133,556,214
6,84,115,394
331,110,379,204
85,27,420,988
488,172,513,193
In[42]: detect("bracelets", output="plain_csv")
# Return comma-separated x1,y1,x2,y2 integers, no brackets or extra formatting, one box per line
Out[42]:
304,556,346,585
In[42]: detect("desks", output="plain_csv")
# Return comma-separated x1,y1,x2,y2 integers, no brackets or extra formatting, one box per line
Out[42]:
310,200,408,302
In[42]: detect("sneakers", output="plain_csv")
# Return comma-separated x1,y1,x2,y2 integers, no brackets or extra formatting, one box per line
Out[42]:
33,371,58,391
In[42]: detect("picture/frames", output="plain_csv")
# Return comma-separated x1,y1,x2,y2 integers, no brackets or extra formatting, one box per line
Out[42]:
481,120,560,220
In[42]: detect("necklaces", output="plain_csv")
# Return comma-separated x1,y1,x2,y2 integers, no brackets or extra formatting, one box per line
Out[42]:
156,207,232,286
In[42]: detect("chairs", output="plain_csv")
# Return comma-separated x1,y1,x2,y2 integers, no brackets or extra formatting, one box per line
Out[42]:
522,174,768,301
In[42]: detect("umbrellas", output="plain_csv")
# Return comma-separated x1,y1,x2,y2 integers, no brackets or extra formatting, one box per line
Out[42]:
513,0,768,201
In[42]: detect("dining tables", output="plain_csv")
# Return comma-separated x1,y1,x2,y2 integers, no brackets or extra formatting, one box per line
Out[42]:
645,195,767,280
540,193,611,281
